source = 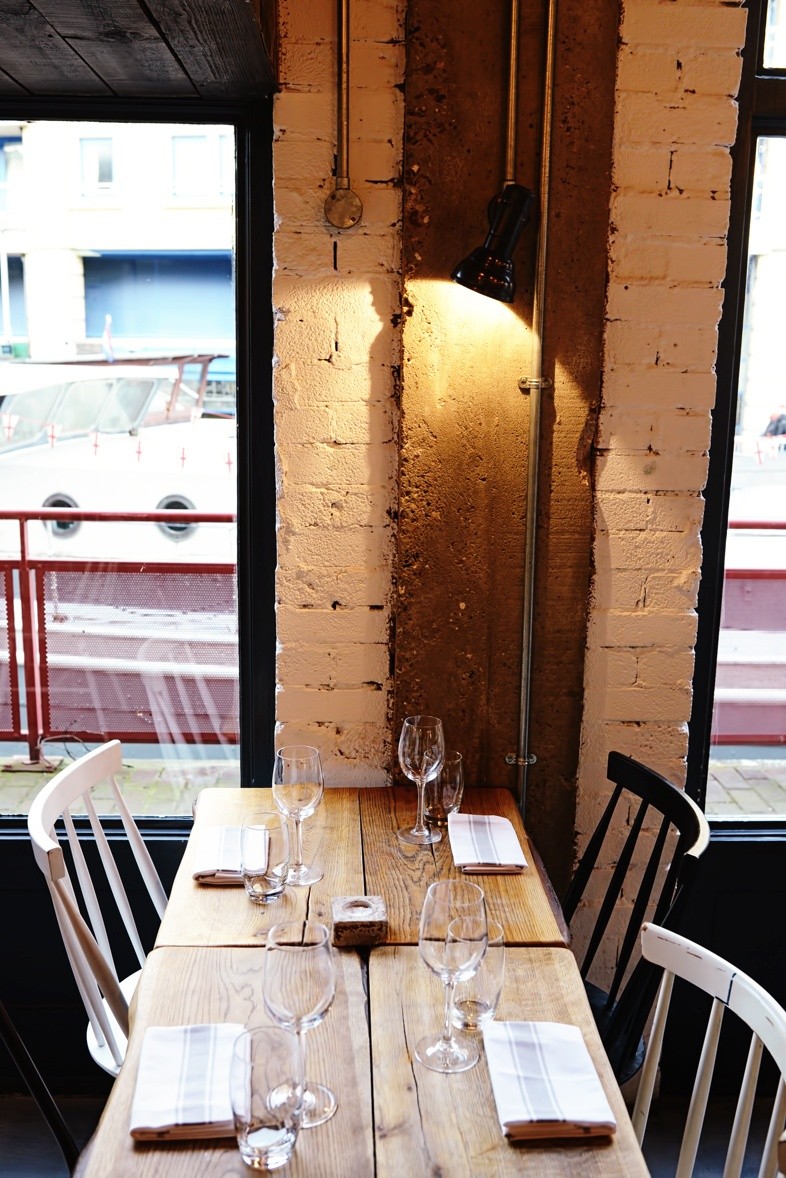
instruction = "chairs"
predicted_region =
[629,921,786,1178]
[562,751,712,1088]
[25,740,169,1081]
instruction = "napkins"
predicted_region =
[478,1020,617,1142]
[193,824,271,885]
[448,812,528,874]
[130,1022,250,1142]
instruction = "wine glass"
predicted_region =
[270,745,326,887]
[411,880,489,1074]
[261,919,339,1129]
[397,715,445,845]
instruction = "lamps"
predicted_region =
[448,184,535,304]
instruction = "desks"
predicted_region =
[69,789,648,1178]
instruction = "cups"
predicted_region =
[229,1026,305,1172]
[444,917,506,1032]
[240,812,290,905]
[419,747,466,827]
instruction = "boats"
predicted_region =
[0,313,786,746]
